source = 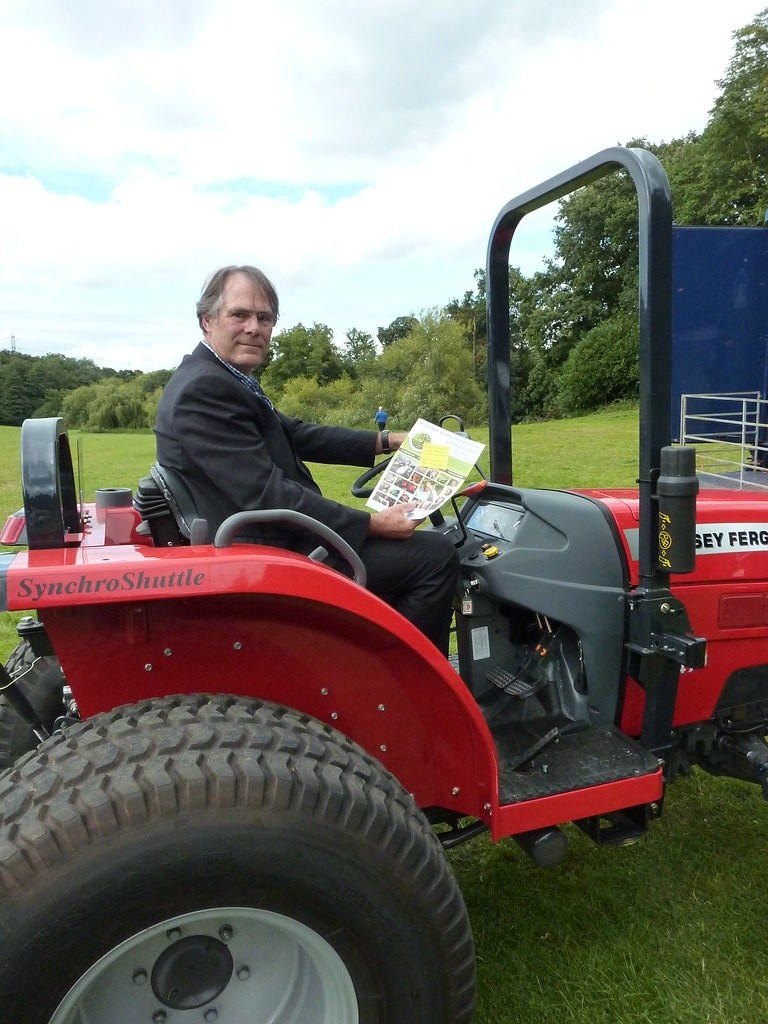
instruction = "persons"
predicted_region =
[152,266,462,664]
[374,405,388,432]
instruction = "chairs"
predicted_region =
[135,458,220,545]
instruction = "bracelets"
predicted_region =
[380,429,391,455]
